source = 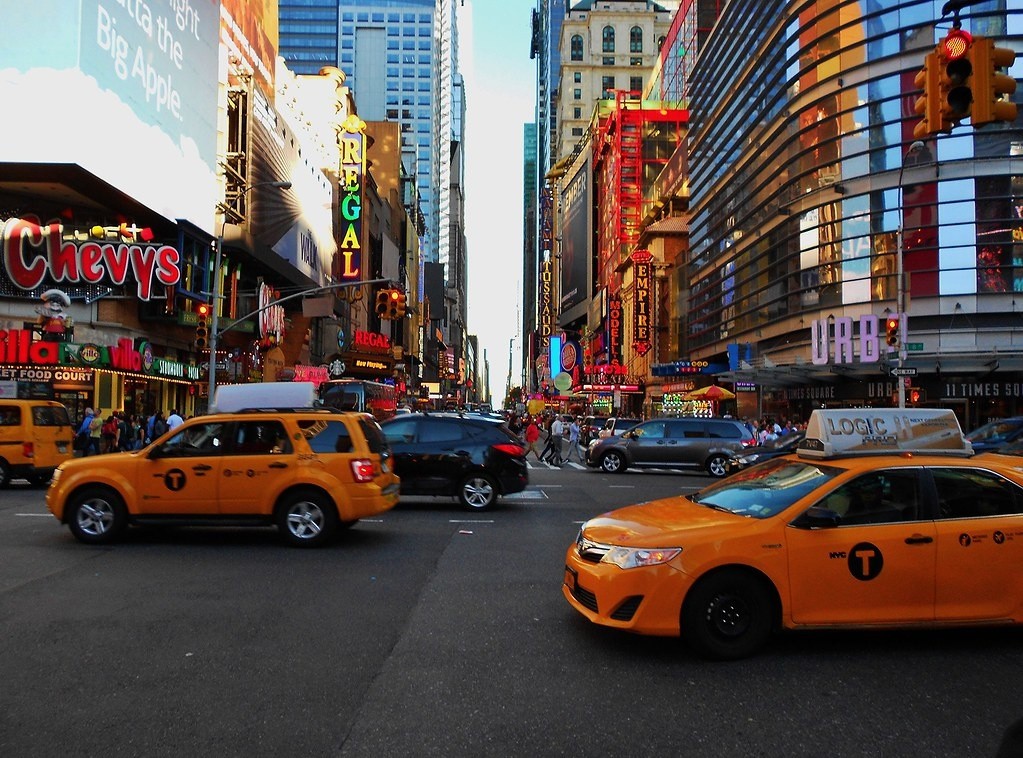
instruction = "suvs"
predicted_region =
[585,417,756,477]
[46,406,400,550]
[380,412,529,511]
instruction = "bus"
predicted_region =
[317,379,397,422]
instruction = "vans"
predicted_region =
[0,398,74,490]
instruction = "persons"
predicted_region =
[967,421,1012,445]
[834,472,906,526]
[70,398,294,459]
[395,397,811,470]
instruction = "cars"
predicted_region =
[724,430,808,476]
[504,409,642,447]
[559,409,1023,659]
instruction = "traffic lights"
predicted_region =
[376,289,408,321]
[913,31,1016,140]
[886,318,899,346]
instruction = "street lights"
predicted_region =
[208,183,292,411]
[898,139,924,410]
